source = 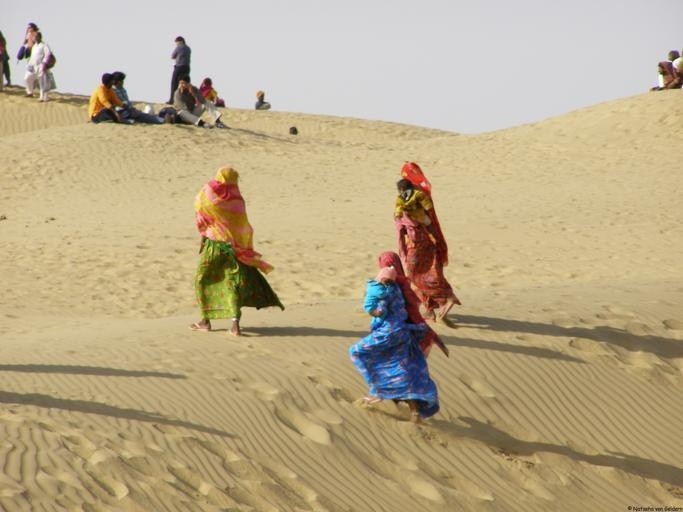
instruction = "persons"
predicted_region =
[189,167,284,335]
[649,50,682,90]
[88,36,231,129]
[255,89,270,110]
[349,161,462,421]
[0,21,55,102]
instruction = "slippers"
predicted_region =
[408,412,422,422]
[420,312,434,319]
[186,323,209,331]
[362,396,381,404]
[225,327,241,335]
[439,297,459,318]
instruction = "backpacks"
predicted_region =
[46,53,55,69]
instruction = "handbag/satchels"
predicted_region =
[37,72,56,93]
